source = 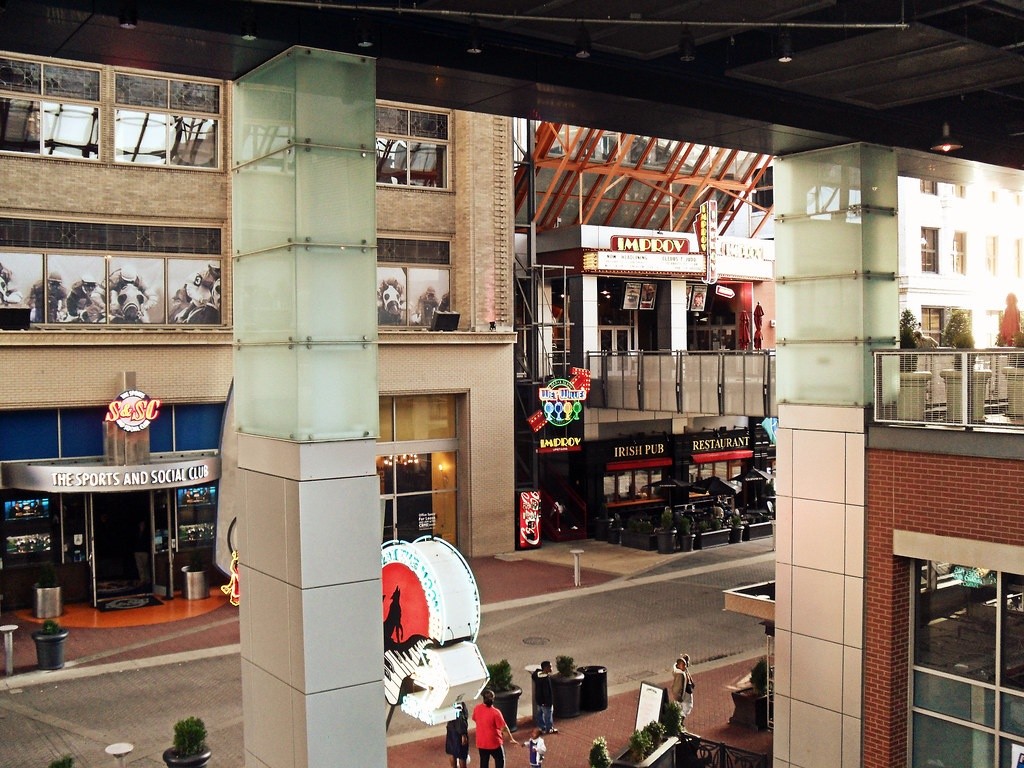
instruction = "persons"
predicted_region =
[915,330,939,349]
[171,260,220,321]
[445,702,470,768]
[0,262,159,323]
[522,727,547,768]
[672,657,693,726]
[684,504,694,515]
[472,689,520,768]
[377,276,450,326]
[532,660,558,733]
[714,502,724,518]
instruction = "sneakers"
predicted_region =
[547,728,558,734]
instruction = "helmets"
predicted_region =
[48,272,61,281]
[120,269,137,282]
[426,287,435,293]
[81,274,98,282]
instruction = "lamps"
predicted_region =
[239,2,258,40]
[726,34,741,69]
[118,0,138,30]
[467,17,482,55]
[676,24,697,64]
[772,26,794,64]
[352,9,376,48]
[573,23,592,60]
[489,320,497,329]
[929,123,966,157]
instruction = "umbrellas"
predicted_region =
[690,475,743,496]
[727,467,776,511]
[753,302,764,348]
[738,306,751,349]
[643,477,706,507]
[1000,292,1021,346]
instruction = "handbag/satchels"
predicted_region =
[686,684,693,694]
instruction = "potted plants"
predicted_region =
[744,514,773,540]
[162,716,212,768]
[592,501,624,544]
[620,519,656,551]
[897,308,932,426]
[480,657,525,733]
[729,514,745,543]
[31,562,63,619]
[695,517,731,550]
[937,307,995,423]
[656,510,678,554]
[181,547,210,600]
[676,516,696,552]
[610,701,702,768]
[551,654,585,719]
[29,617,70,671]
[1002,330,1024,417]
[728,656,775,732]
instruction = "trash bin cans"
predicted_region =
[578,664,607,712]
